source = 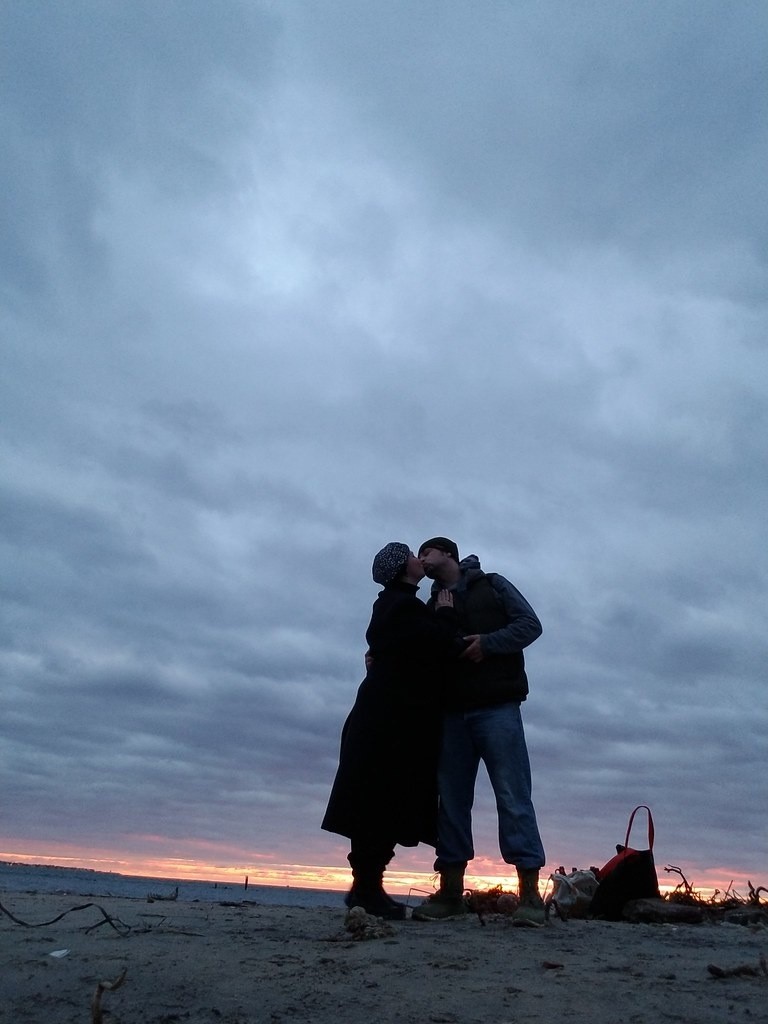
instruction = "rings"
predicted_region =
[445,599,450,601]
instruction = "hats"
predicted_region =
[372,542,410,587]
[418,537,460,563]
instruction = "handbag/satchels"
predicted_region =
[544,870,600,920]
[589,806,660,913]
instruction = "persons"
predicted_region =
[365,538,549,927]
[319,542,456,920]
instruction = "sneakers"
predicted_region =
[413,888,467,922]
[512,896,547,927]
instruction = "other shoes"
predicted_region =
[345,873,406,920]
